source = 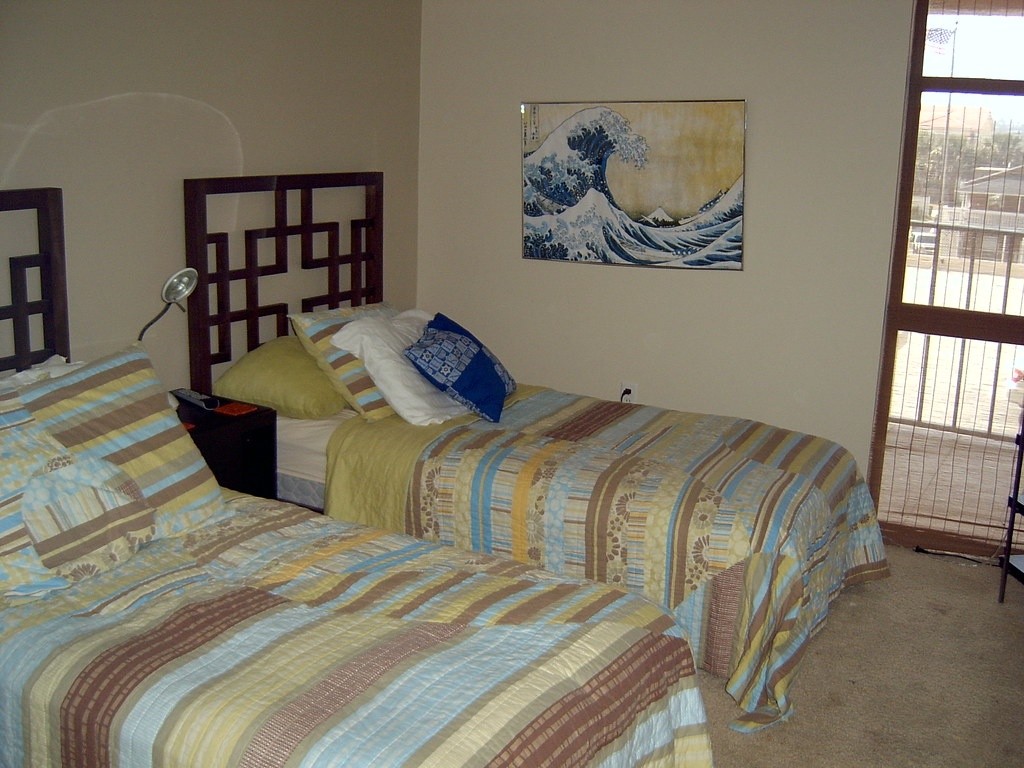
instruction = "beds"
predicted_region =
[184,171,891,734]
[0,187,715,768]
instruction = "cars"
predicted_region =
[910,232,935,255]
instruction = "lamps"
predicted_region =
[137,267,199,342]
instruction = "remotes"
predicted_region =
[173,388,220,410]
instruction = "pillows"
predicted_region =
[402,312,517,423]
[330,308,473,426]
[213,336,349,421]
[284,301,398,423]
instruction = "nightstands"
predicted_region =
[170,387,277,502]
